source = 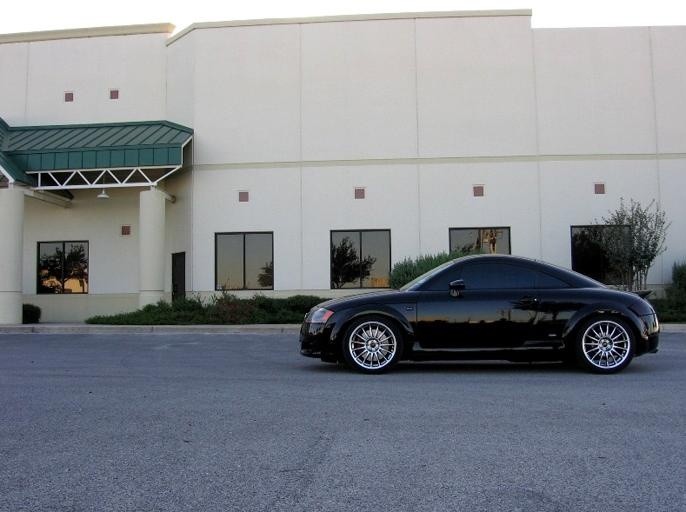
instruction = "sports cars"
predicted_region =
[297,253,663,374]
